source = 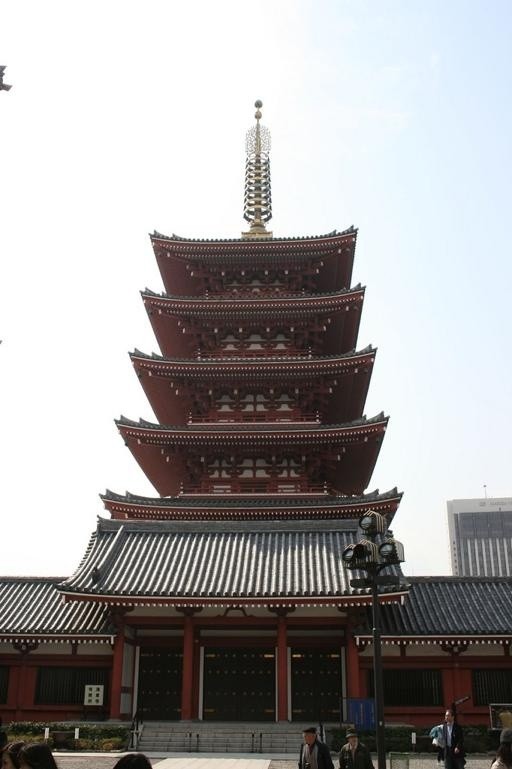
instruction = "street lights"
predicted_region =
[342,510,405,769]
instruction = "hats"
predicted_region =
[302,726,317,735]
[345,727,359,738]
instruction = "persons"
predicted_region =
[435,721,446,767]
[498,710,511,769]
[0,730,59,769]
[491,743,511,769]
[338,728,375,769]
[297,727,334,769]
[441,710,464,769]
[112,753,152,768]
